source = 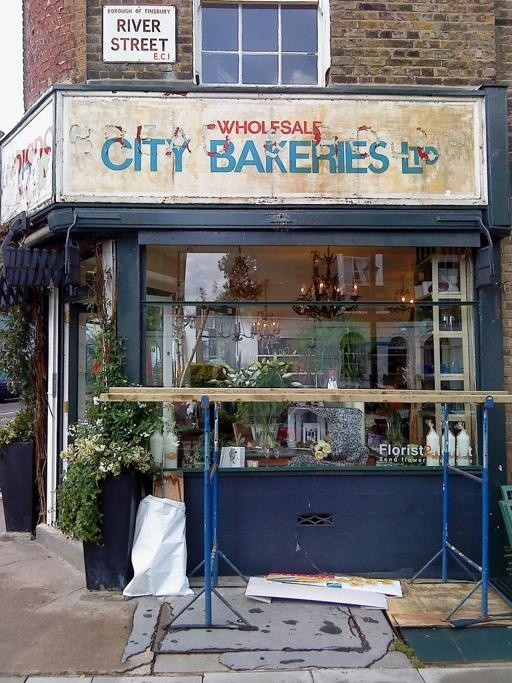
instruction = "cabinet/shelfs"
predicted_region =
[414,252,472,449]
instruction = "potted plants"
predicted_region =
[2,292,36,532]
[303,320,362,388]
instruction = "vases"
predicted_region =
[385,414,404,443]
[81,461,143,592]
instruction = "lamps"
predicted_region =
[218,245,362,343]
[385,272,416,314]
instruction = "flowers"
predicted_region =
[37,293,167,549]
[206,348,302,447]
[371,402,405,444]
